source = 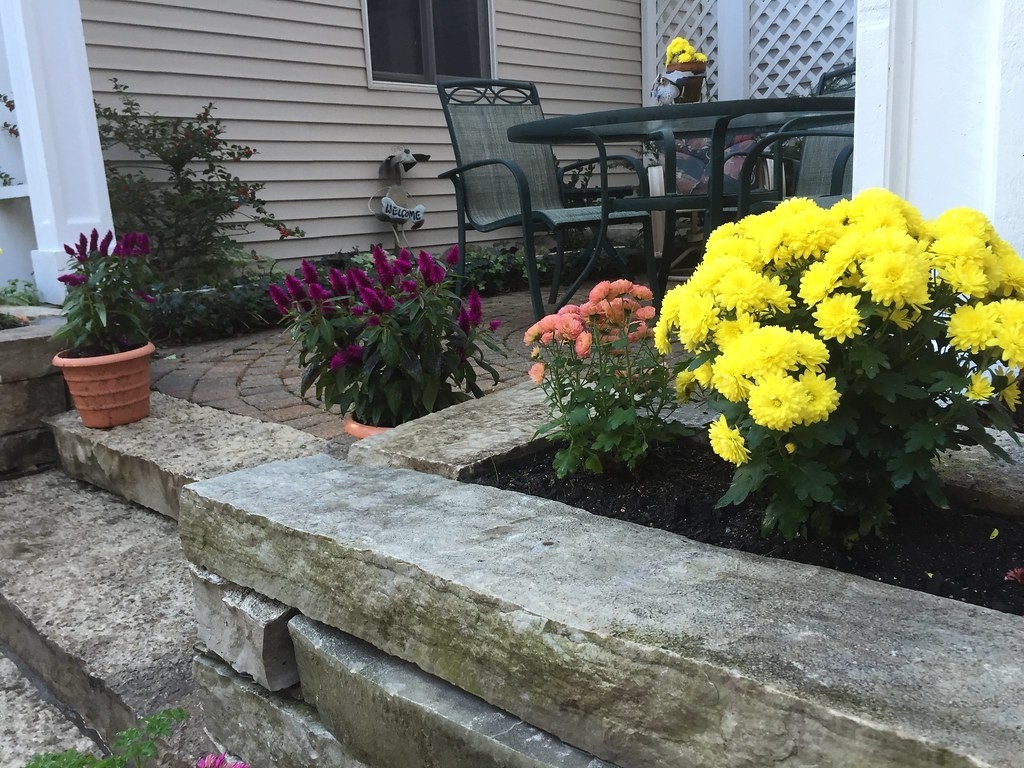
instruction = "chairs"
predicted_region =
[436,77,659,329]
[701,62,856,254]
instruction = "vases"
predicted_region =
[344,392,473,440]
[650,62,706,104]
[52,341,156,428]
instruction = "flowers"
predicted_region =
[267,241,512,430]
[48,228,159,356]
[665,37,707,66]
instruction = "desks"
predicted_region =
[507,97,853,328]
